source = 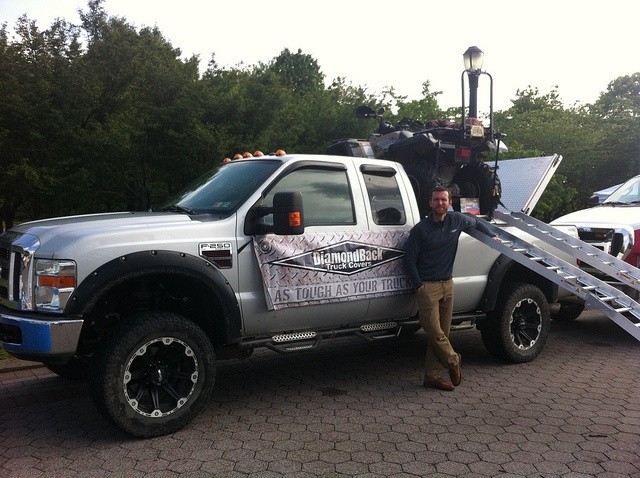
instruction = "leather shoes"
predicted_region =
[450,353,461,385]
[424,378,455,391]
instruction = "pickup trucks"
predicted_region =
[552,175,639,324]
[0,150,586,438]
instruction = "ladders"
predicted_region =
[464,213,640,344]
[493,210,639,292]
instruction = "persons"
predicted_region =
[402,185,501,393]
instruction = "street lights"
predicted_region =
[462,46,494,142]
